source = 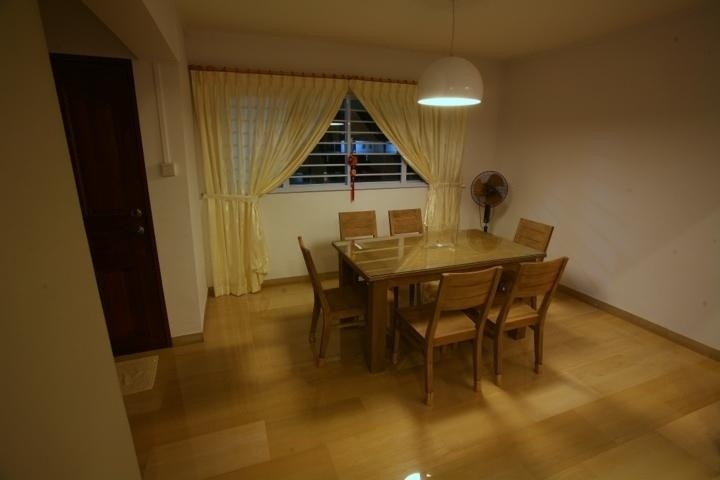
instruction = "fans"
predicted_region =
[470,170,509,231]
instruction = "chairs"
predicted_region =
[389,265,502,407]
[499,218,555,313]
[338,208,443,307]
[297,236,400,365]
[447,256,569,386]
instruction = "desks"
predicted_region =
[332,228,547,375]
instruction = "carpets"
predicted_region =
[116,353,159,396]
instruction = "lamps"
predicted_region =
[415,1,485,109]
